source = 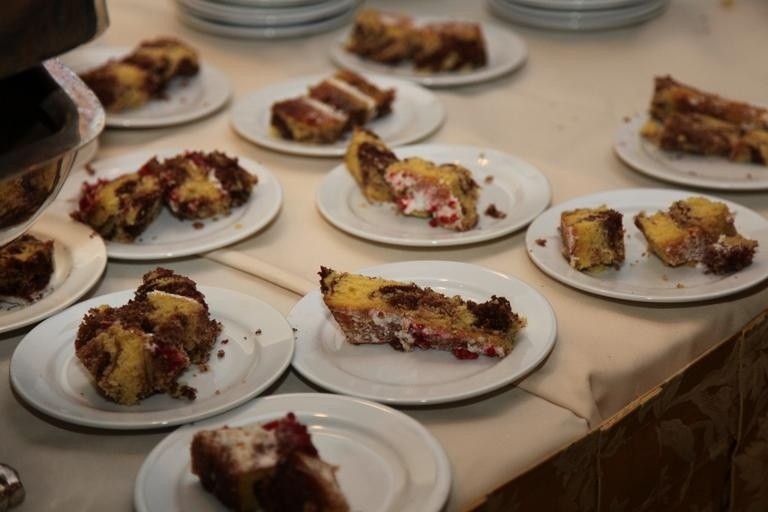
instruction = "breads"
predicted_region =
[0,234,54,301]
[344,122,505,233]
[344,10,488,73]
[68,147,257,243]
[271,65,396,146]
[189,412,349,510]
[639,72,764,178]
[78,34,199,114]
[316,265,527,362]
[560,204,625,272]
[633,196,759,276]
[73,266,228,405]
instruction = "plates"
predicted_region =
[330,18,532,91]
[230,72,447,158]
[287,259,559,407]
[175,2,358,41]
[0,211,107,335]
[54,45,231,132]
[8,285,298,430]
[525,188,768,305]
[491,1,666,34]
[616,101,766,191]
[59,148,285,260]
[314,145,552,251]
[133,389,451,509]
